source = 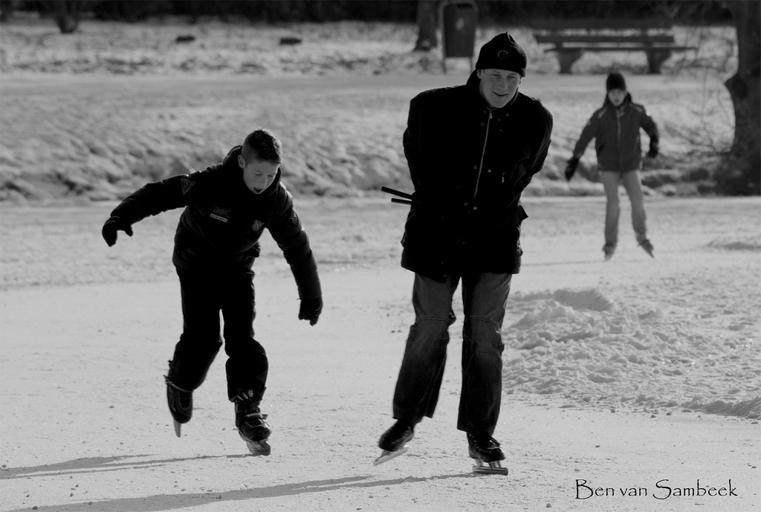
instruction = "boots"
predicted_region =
[602,243,614,253]
[641,240,652,251]
[235,403,271,439]
[379,423,414,451]
[469,435,503,461]
[167,382,192,422]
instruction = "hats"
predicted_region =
[607,73,625,90]
[478,33,526,77]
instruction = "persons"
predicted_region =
[379,33,552,460]
[564,72,659,253]
[102,129,322,438]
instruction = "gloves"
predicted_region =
[647,149,657,158]
[299,297,321,325]
[565,160,575,180]
[102,216,132,246]
